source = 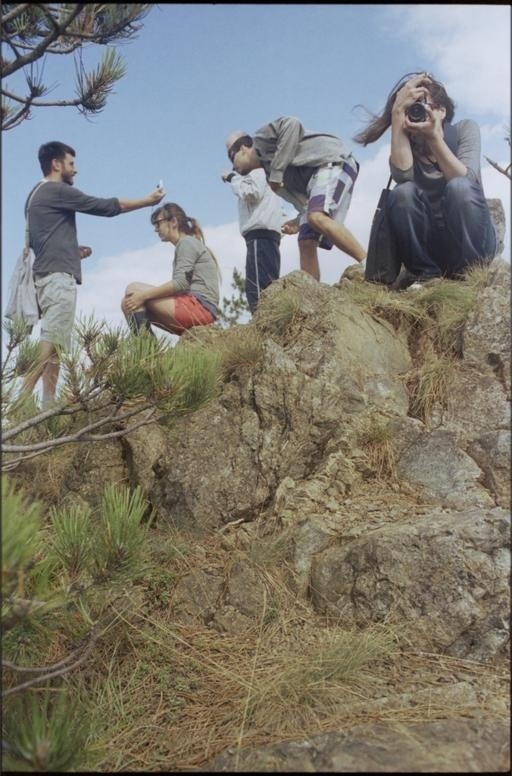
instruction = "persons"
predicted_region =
[8,139,167,420]
[225,115,368,284]
[220,168,286,316]
[352,71,496,293]
[121,201,220,355]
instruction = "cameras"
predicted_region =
[407,95,434,122]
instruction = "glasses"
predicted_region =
[152,216,171,228]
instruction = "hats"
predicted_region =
[225,130,247,151]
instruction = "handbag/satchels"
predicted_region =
[364,189,401,284]
[4,248,39,326]
